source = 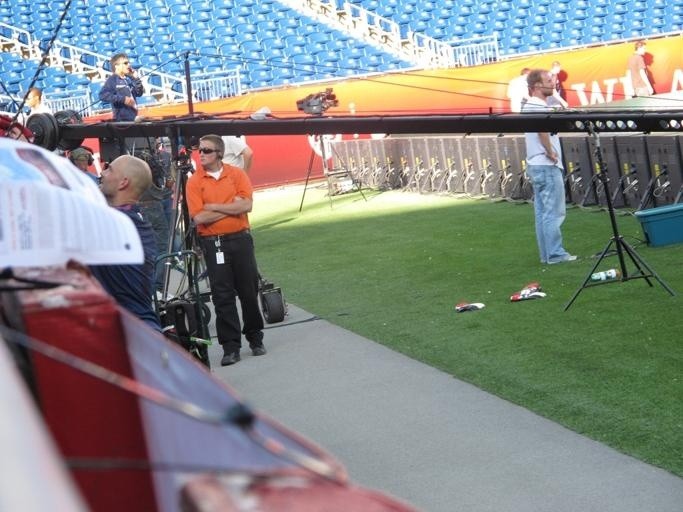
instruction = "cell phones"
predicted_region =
[129,68,132,73]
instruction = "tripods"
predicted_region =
[300,135,366,210]
[564,124,676,312]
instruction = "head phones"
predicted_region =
[69,146,95,164]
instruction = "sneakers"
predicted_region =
[540,255,578,265]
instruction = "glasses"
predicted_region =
[199,147,220,154]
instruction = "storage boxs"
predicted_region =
[634,203,683,247]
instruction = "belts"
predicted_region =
[203,230,249,241]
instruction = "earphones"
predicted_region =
[218,156,222,160]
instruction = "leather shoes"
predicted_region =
[222,351,240,366]
[252,338,266,355]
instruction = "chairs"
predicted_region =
[2,1,420,100]
[1,49,161,113]
[321,0,683,66]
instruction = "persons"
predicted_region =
[98,52,143,119]
[627,41,654,98]
[518,67,577,266]
[7,88,176,334]
[506,68,531,114]
[183,133,266,366]
[217,133,253,173]
[543,60,571,109]
